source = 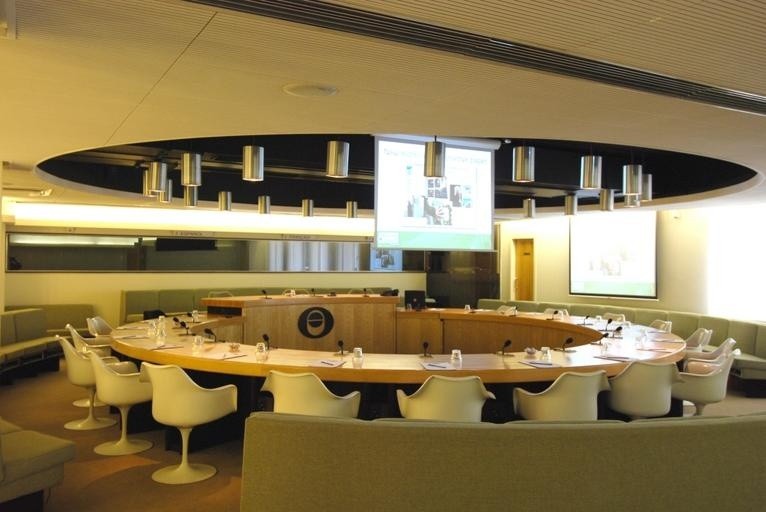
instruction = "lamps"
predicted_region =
[142,135,270,214]
[346,201,357,219]
[423,134,446,178]
[511,139,652,218]
[302,199,313,217]
[324,134,350,179]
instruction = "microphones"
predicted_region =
[336,339,347,356]
[612,326,623,337]
[262,289,269,297]
[311,286,315,295]
[562,336,575,351]
[174,316,181,325]
[605,318,613,330]
[204,327,217,342]
[180,320,188,332]
[598,333,609,341]
[421,341,430,357]
[262,332,271,350]
[362,287,368,295]
[501,339,513,355]
[583,315,589,324]
[550,311,559,319]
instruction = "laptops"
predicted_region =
[405,288,428,308]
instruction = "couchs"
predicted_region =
[477,298,766,384]
[0,304,96,512]
[237,409,765,510]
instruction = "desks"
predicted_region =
[110,292,689,450]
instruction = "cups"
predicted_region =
[539,347,550,363]
[406,304,411,311]
[600,346,608,359]
[557,311,563,320]
[451,349,462,363]
[148,310,267,360]
[351,348,364,368]
[595,316,601,325]
[464,304,470,313]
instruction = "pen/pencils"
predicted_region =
[530,362,552,365]
[608,356,629,359]
[321,361,333,365]
[428,363,446,368]
